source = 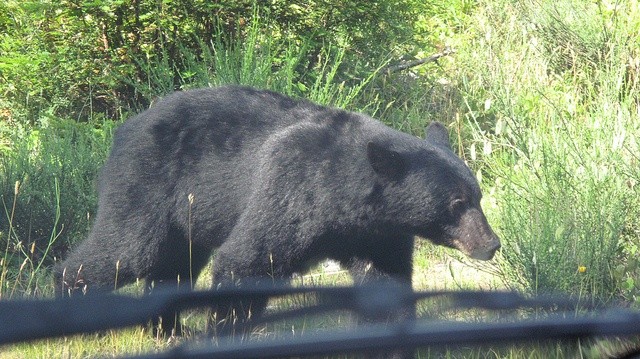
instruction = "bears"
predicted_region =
[48,84,502,358]
